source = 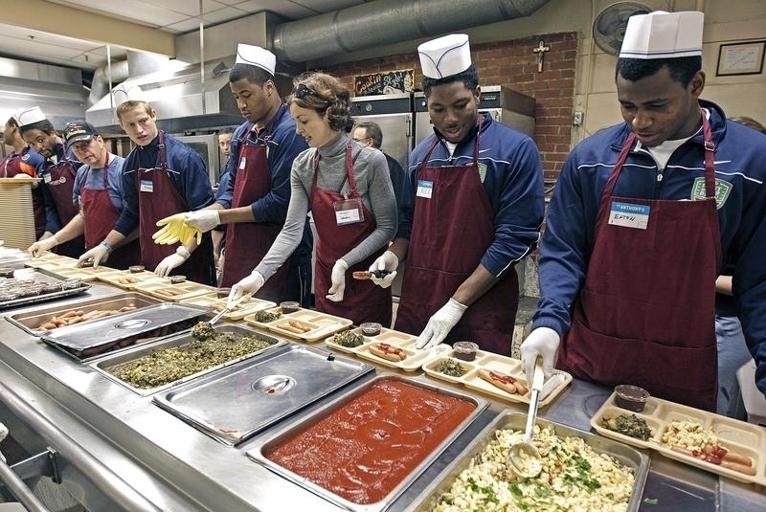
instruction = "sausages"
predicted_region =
[210,302,239,314]
[35,301,189,359]
[476,369,528,395]
[118,276,135,284]
[370,341,409,362]
[678,446,757,475]
[280,321,310,335]
[156,288,180,299]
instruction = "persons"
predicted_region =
[15,106,86,260]
[152,43,312,306]
[227,71,398,330]
[368,32,546,357]
[0,109,46,241]
[714,116,766,419]
[27,121,141,271]
[519,11,766,415]
[211,126,236,288]
[73,85,217,288]
[351,120,406,210]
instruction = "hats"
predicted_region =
[114,82,146,107]
[618,9,707,60]
[235,43,277,77]
[14,105,47,127]
[62,120,99,152]
[417,33,472,80]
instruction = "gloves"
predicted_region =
[152,210,220,246]
[325,260,346,302]
[518,327,560,389]
[227,271,264,310]
[29,236,58,258]
[414,298,468,350]
[368,250,399,289]
[154,245,192,278]
[76,241,112,268]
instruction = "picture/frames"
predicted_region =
[716,40,766,76]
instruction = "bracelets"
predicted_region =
[101,241,113,252]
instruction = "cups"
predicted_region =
[452,339,480,361]
[78,259,95,268]
[360,321,382,337]
[216,287,235,299]
[169,274,185,284]
[280,300,300,314]
[616,382,651,412]
[129,265,145,274]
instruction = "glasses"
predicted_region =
[291,83,327,101]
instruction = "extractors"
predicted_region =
[83,12,307,140]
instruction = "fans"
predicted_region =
[592,0,653,56]
